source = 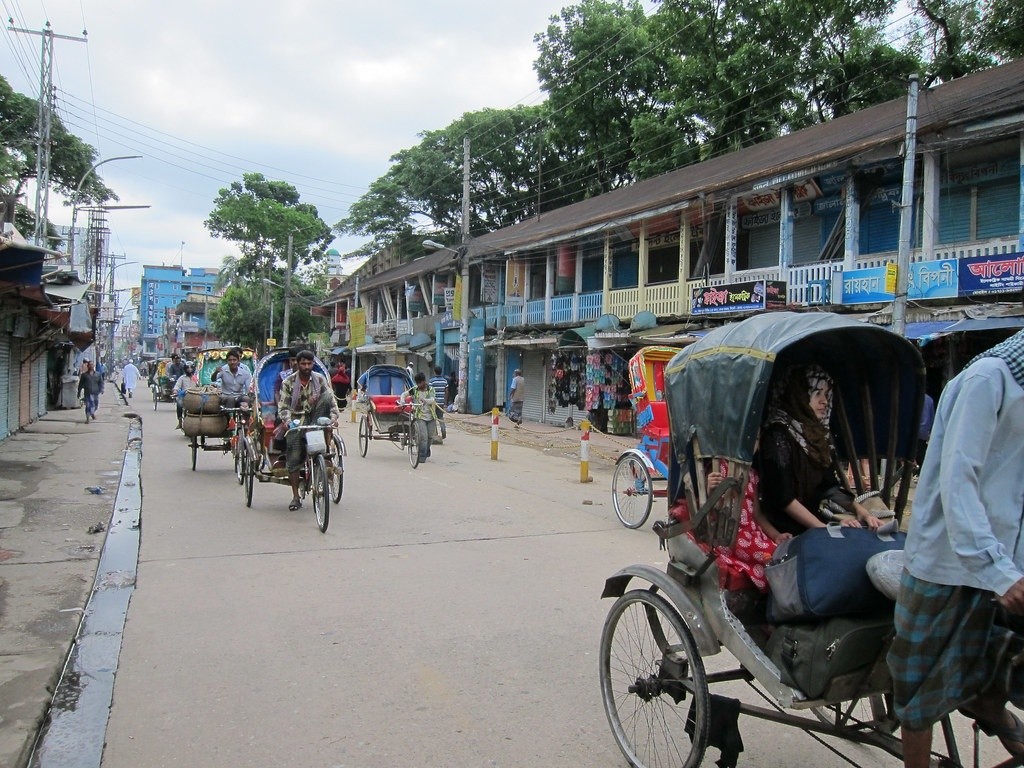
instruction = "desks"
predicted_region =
[644,401,670,438]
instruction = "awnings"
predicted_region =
[886,314,1024,344]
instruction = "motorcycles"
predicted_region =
[170,390,193,437]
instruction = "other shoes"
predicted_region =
[289,500,301,508]
[419,456,426,463]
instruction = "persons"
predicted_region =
[428,366,449,439]
[399,372,436,464]
[507,369,525,428]
[99,347,350,512]
[668,425,794,634]
[406,362,415,381]
[752,361,884,537]
[887,323,1024,768]
[77,361,104,424]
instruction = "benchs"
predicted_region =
[368,395,412,413]
[259,402,275,447]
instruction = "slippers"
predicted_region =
[958,704,1024,766]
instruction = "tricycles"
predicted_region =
[357,364,425,469]
[597,307,1024,768]
[610,345,671,530]
[150,356,180,410]
[137,360,148,378]
[231,347,347,533]
[187,340,255,485]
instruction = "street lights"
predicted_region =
[421,240,469,413]
[262,278,289,347]
